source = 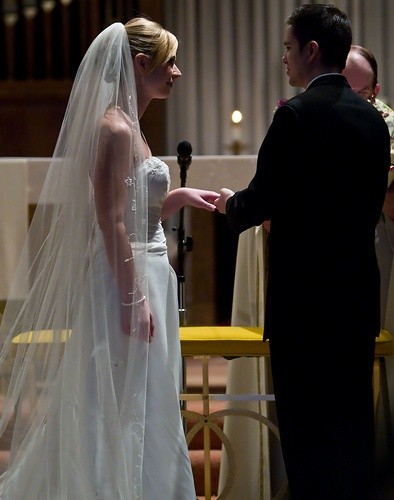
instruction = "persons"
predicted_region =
[213,3,394,500]
[0,16,220,500]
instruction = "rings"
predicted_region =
[132,328,137,333]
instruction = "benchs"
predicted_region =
[9,326,394,500]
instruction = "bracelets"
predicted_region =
[121,296,145,308]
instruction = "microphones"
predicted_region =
[176,141,193,179]
